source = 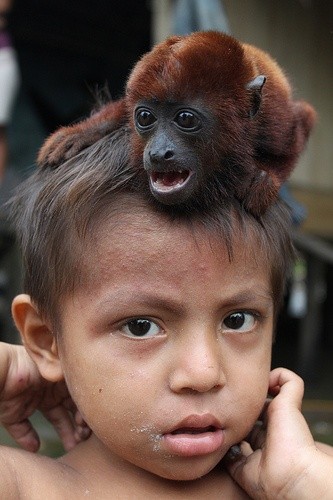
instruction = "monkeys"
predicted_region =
[38,30,316,216]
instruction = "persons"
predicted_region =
[0,122,333,500]
[0,1,20,188]
